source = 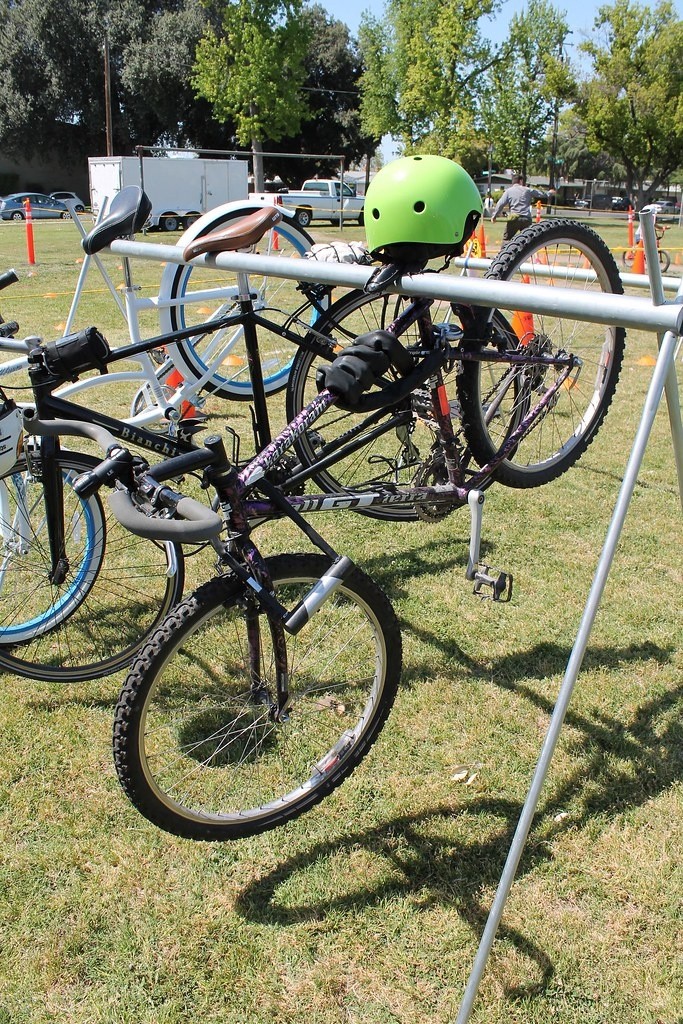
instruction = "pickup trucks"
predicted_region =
[248,179,366,226]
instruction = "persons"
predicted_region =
[491,174,556,257]
[485,196,494,207]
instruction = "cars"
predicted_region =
[47,192,85,212]
[1,192,72,221]
[651,201,680,214]
[612,197,631,211]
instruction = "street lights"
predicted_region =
[546,29,573,214]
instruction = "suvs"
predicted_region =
[575,193,613,211]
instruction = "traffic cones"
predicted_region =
[541,254,547,264]
[504,273,538,342]
[630,241,645,277]
[463,223,487,260]
[673,253,682,265]
[583,258,592,270]
[160,342,207,423]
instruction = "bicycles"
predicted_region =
[623,224,671,272]
[0,185,628,841]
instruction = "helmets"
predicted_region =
[0,399,23,475]
[363,154,482,259]
[643,205,657,216]
